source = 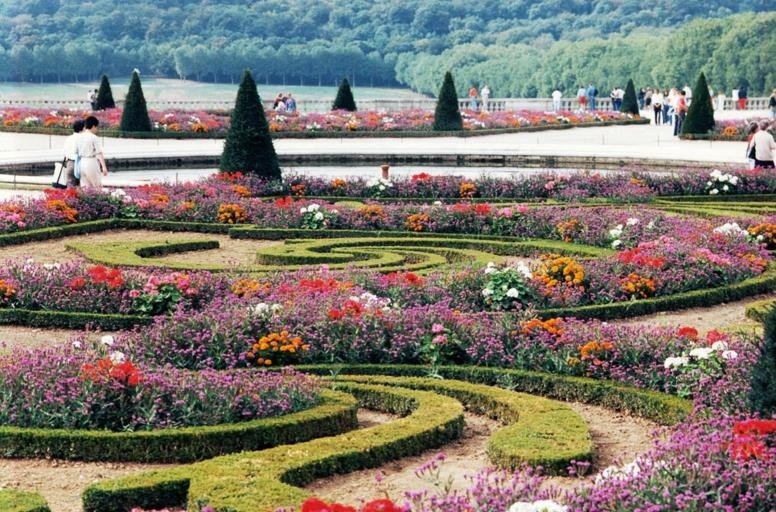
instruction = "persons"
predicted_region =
[768,88,776,118]
[746,120,776,169]
[470,84,490,112]
[273,92,296,112]
[87,89,98,110]
[732,86,747,110]
[552,83,714,136]
[65,116,108,190]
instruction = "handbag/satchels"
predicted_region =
[51,157,67,189]
[748,135,756,160]
